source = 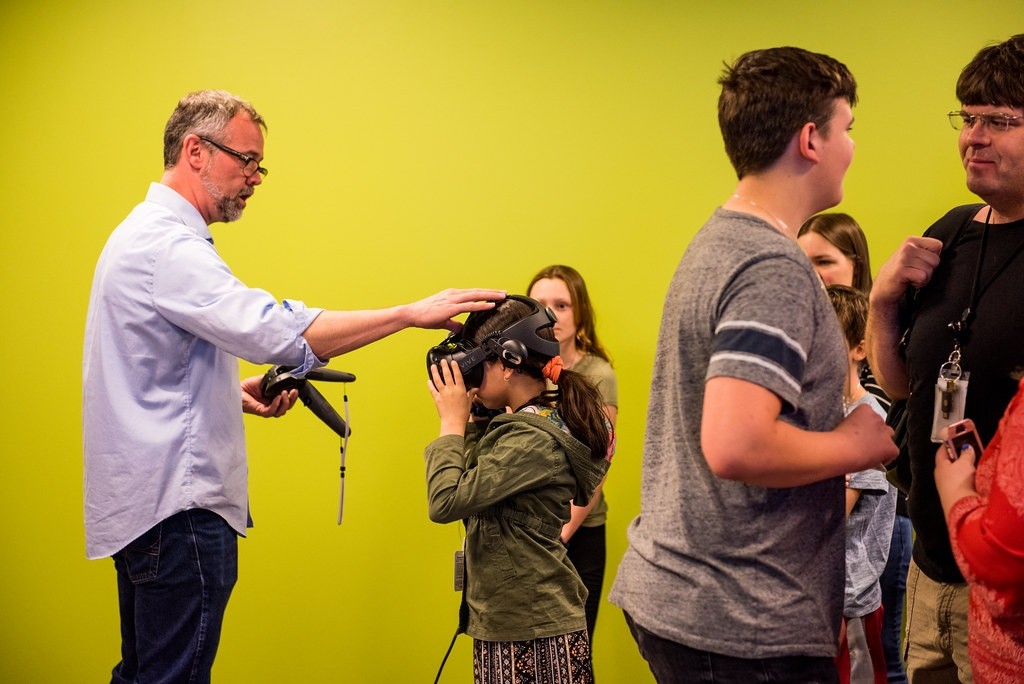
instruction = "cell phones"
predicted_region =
[939,418,985,468]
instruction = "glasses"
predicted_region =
[181,136,268,182]
[947,110,1024,135]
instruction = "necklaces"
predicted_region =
[845,381,860,408]
[732,193,852,398]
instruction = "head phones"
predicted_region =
[458,294,560,372]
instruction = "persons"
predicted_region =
[862,32,1024,684]
[85,89,509,684]
[797,213,914,684]
[607,47,901,684]
[424,294,614,684]
[935,376,1024,684]
[525,265,619,641]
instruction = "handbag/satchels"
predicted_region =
[880,202,986,500]
